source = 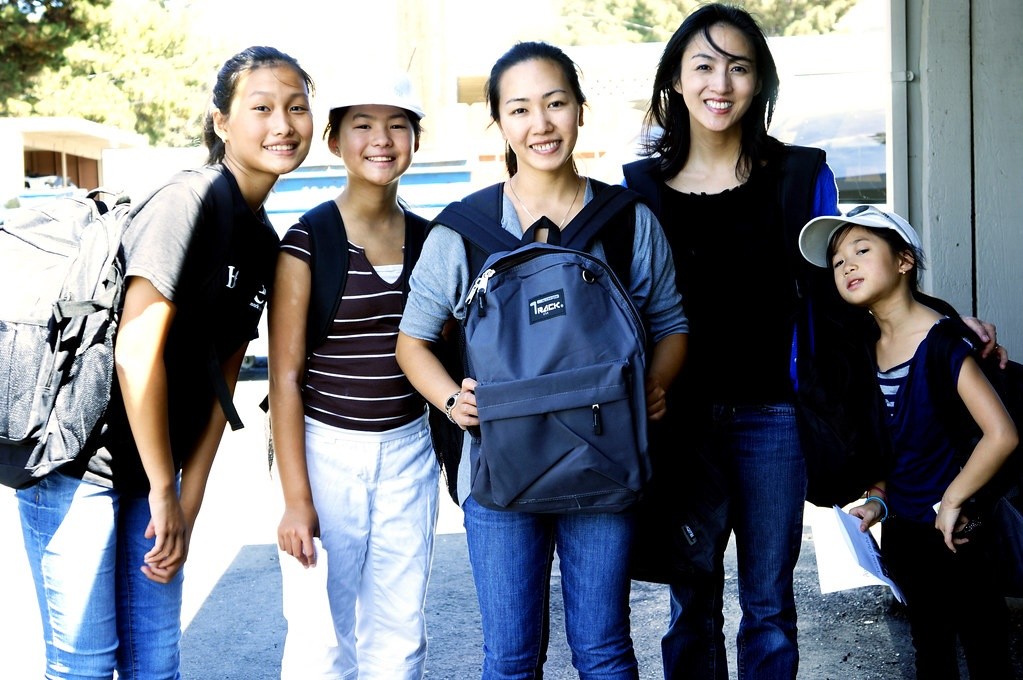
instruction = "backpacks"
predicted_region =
[0,164,220,490]
[425,184,653,514]
[926,319,1023,505]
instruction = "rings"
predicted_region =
[994,344,1000,352]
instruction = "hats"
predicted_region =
[799,204,924,282]
[324,61,425,122]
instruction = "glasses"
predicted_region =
[843,205,912,244]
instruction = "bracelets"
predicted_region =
[872,485,887,498]
[865,496,888,522]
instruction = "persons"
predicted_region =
[394,39,687,680]
[267,78,459,680]
[793,204,1023,680]
[613,5,1007,680]
[11,48,318,680]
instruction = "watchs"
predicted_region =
[445,392,461,425]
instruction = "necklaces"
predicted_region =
[509,177,581,230]
